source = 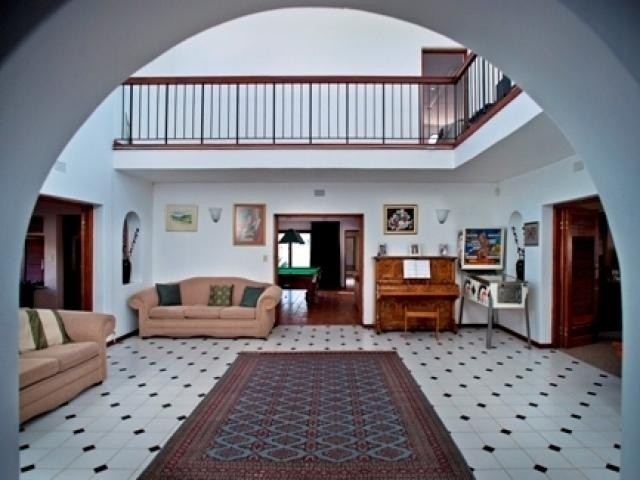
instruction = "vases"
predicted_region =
[123,259,130,283]
[516,260,524,281]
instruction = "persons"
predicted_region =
[463,279,472,297]
[477,286,488,304]
[476,229,496,265]
[240,213,262,241]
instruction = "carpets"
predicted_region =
[136,351,475,480]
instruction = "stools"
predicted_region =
[404,306,440,340]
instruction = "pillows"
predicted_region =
[208,283,234,306]
[19,307,72,354]
[156,283,182,306]
[240,287,264,307]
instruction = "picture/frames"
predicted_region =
[524,221,538,246]
[166,204,197,231]
[384,204,418,234]
[232,203,266,246]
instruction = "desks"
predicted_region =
[458,273,531,350]
[278,267,322,308]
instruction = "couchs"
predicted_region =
[128,277,283,341]
[19,309,116,425]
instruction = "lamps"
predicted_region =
[278,228,305,266]
[436,209,450,224]
[209,208,222,223]
